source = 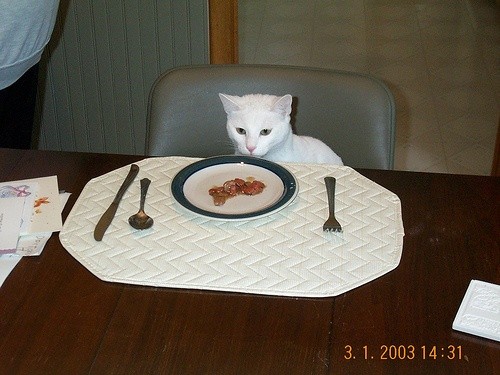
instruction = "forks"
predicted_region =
[323,177,342,233]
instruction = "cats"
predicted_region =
[218,92,343,164]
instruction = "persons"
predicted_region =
[0,0,59,149]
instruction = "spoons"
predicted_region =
[128,178,153,230]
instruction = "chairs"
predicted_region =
[143,63,394,170]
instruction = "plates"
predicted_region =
[170,154,299,222]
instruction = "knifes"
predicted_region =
[94,164,139,241]
